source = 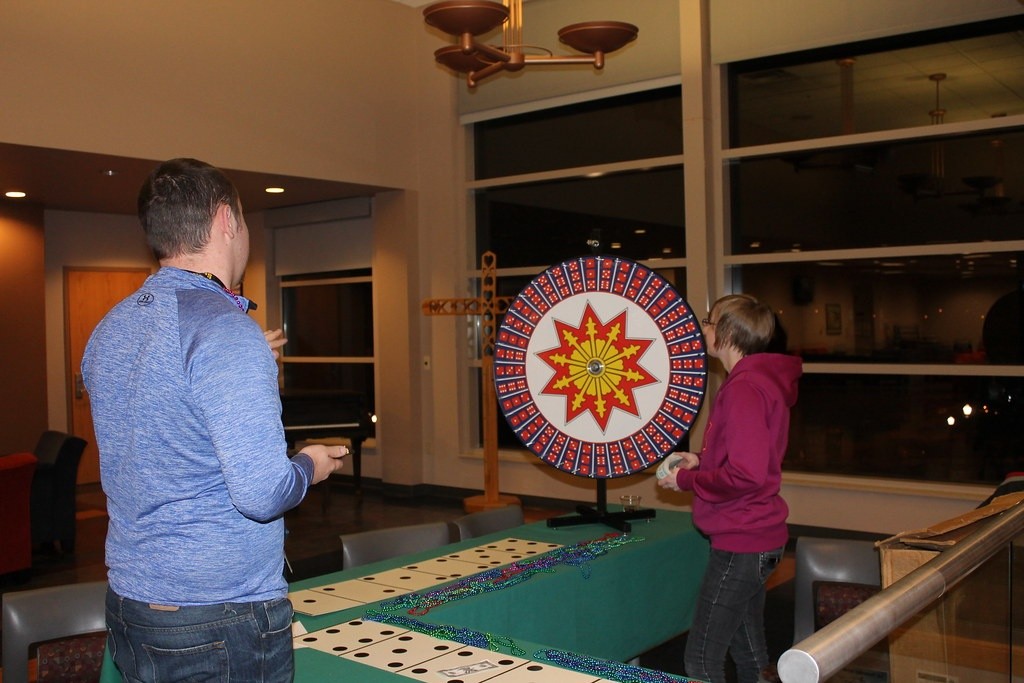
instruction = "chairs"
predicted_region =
[340,506,525,570]
[2,579,109,683]
[794,537,890,683]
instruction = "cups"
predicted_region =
[620,495,642,513]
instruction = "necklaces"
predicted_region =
[224,288,245,312]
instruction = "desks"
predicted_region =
[100,502,714,682]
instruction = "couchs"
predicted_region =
[0,431,87,586]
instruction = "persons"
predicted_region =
[81,158,346,683]
[658,294,802,683]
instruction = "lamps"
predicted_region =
[423,0,639,88]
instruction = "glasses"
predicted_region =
[702,319,716,327]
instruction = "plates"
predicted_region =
[287,446,354,459]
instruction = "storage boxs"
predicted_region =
[875,490,1024,683]
